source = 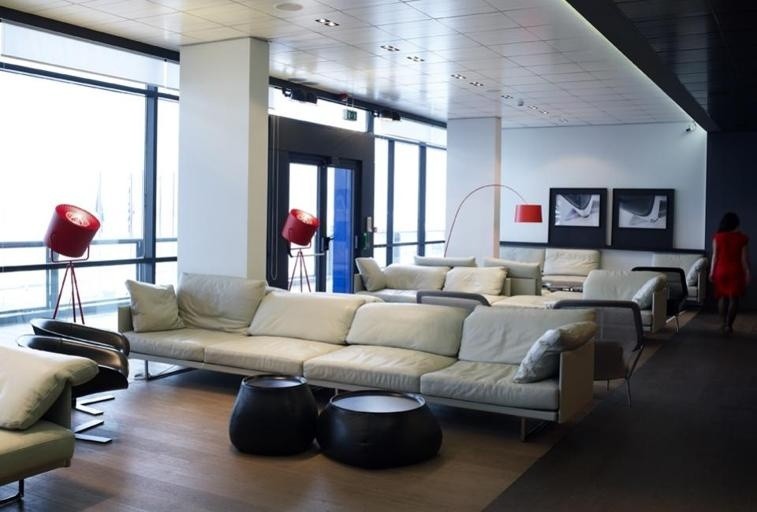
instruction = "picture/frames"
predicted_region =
[611,188,677,250]
[548,187,608,249]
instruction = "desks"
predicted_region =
[480,314,757,512]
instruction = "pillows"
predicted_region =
[0,347,99,431]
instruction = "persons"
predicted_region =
[709,212,750,337]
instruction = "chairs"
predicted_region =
[17,318,131,446]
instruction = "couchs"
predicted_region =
[0,347,74,507]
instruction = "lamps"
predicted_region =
[444,184,542,258]
[282,209,320,292]
[43,204,101,324]
